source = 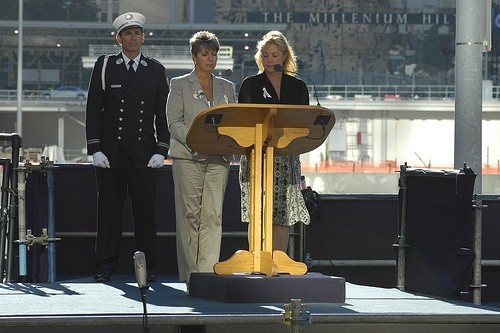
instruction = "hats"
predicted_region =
[113,12,146,35]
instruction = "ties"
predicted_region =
[128,60,135,80]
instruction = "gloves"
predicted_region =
[92,150,110,168]
[147,153,165,168]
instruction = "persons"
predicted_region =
[86,12,171,283]
[238,30,311,253]
[165,31,237,284]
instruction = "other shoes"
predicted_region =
[147,271,155,281]
[94,263,113,281]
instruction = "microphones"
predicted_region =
[133,251,147,291]
[274,64,320,105]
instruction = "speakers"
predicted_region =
[396,163,476,302]
